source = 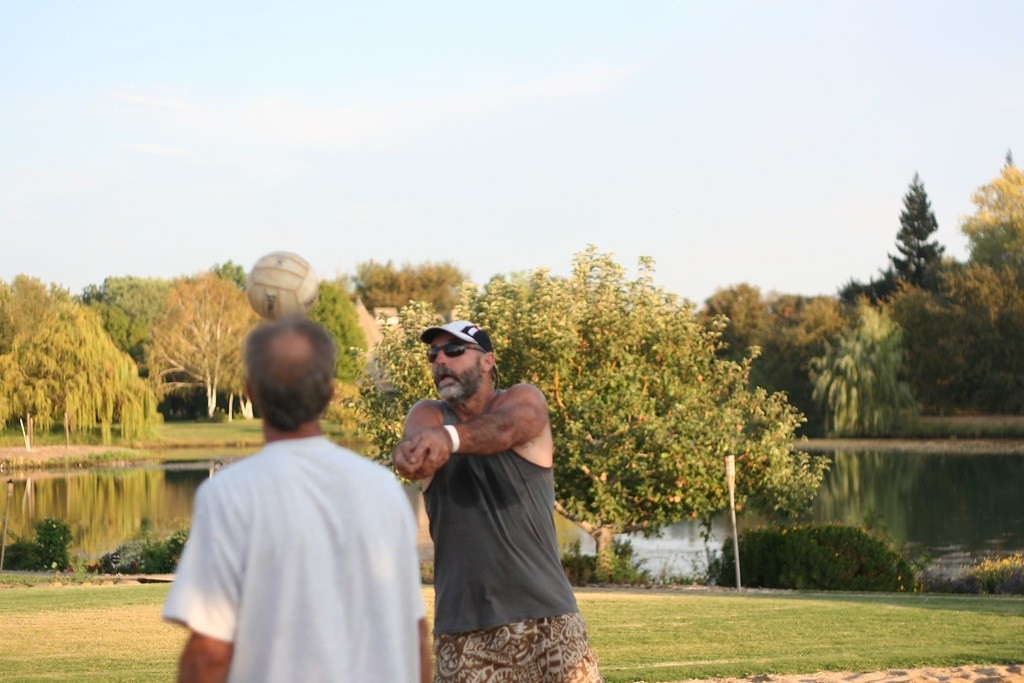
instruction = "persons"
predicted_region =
[391,318,603,683]
[164,314,432,683]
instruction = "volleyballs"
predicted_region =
[245,250,322,322]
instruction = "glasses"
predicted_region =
[426,342,487,363]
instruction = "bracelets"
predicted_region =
[443,425,461,454]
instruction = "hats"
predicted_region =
[419,320,493,354]
